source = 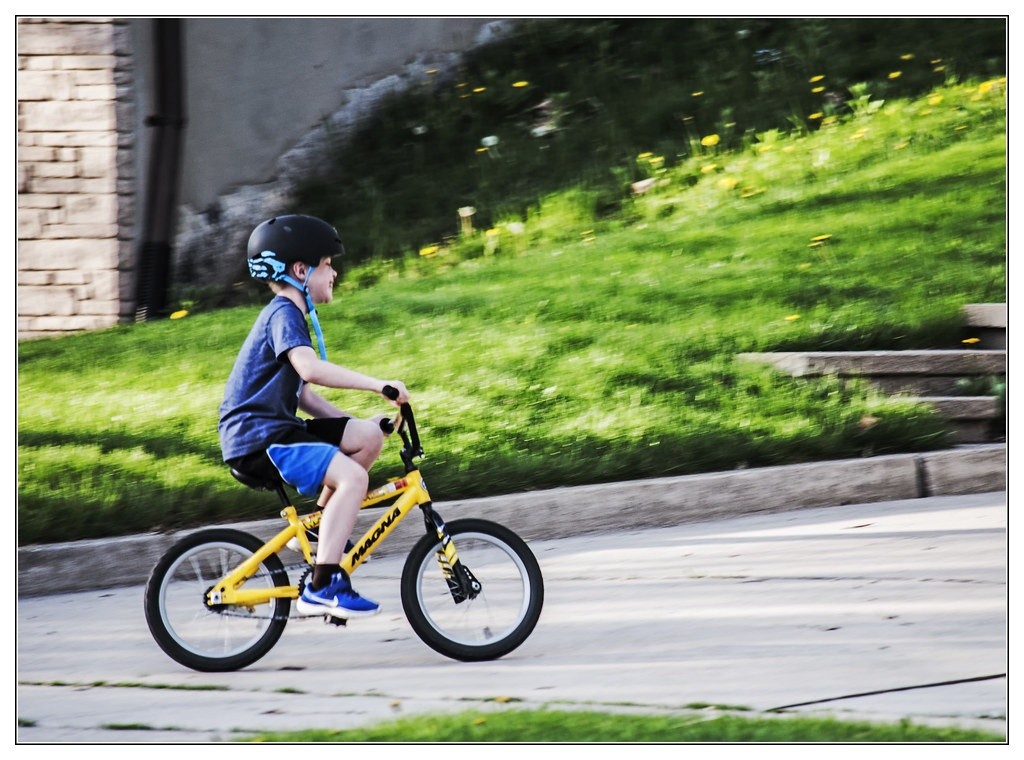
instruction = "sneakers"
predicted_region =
[286,529,373,567]
[295,573,380,620]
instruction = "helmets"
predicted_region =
[244,213,346,279]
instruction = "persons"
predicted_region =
[217,214,411,618]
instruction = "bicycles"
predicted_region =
[142,384,544,675]
